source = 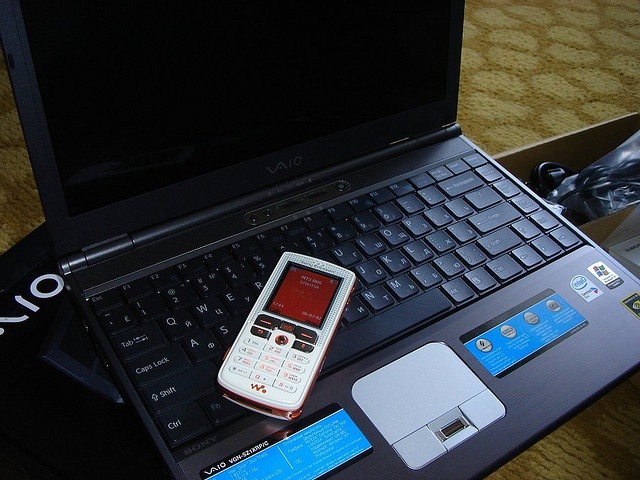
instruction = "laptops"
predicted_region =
[0,0,640,480]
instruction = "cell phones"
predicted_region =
[214,251,357,422]
